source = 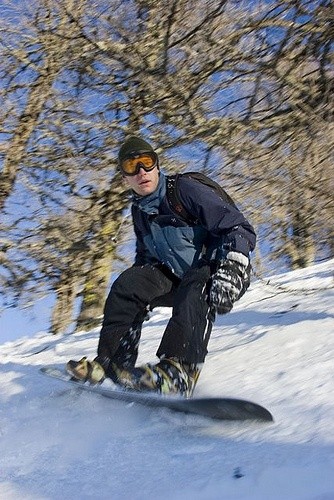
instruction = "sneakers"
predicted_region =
[66,357,132,391]
[118,357,201,399]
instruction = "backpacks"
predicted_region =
[164,172,253,317]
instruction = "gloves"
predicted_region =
[199,250,251,315]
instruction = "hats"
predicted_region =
[118,137,160,178]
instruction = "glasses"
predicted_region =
[119,150,158,176]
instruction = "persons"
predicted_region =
[67,137,256,398]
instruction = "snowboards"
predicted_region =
[38,368,272,422]
